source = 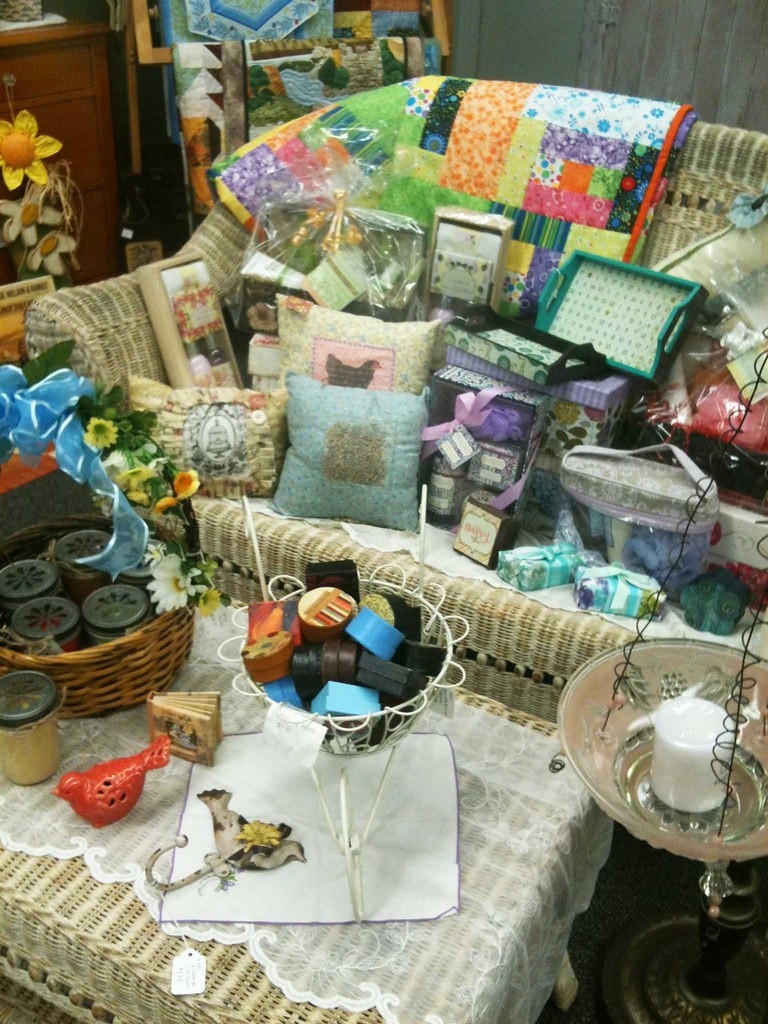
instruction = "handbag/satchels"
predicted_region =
[558,442,720,602]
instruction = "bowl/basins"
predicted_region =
[557,638,768,863]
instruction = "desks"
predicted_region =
[1,0,122,292]
[0,556,611,1024]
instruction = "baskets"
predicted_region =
[0,512,196,718]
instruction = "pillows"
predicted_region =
[128,375,286,499]
[268,366,429,536]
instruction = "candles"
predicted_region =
[649,698,737,816]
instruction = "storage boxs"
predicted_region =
[441,324,669,625]
[704,487,768,613]
[136,252,245,394]
[419,205,514,318]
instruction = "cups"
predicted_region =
[0,559,62,623]
[82,584,150,645]
[12,597,80,650]
[112,539,167,595]
[54,530,111,605]
[0,672,66,785]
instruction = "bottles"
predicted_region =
[116,175,170,274]
[426,410,469,526]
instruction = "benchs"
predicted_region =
[30,75,768,723]
